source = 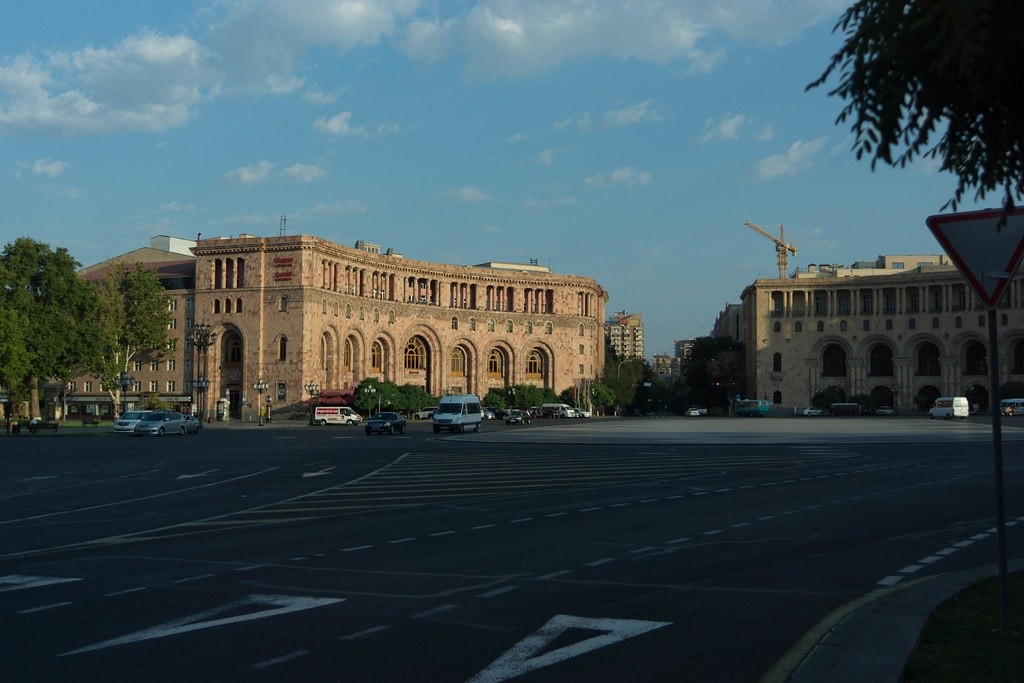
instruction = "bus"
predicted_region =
[1000,398,1024,416]
[734,400,770,418]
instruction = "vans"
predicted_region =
[531,402,590,419]
[428,394,482,434]
[314,406,363,426]
[928,396,969,419]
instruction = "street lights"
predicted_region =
[305,380,320,426]
[192,374,211,421]
[115,371,136,414]
[252,377,269,426]
[618,359,632,379]
[365,384,376,420]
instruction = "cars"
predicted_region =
[111,410,154,434]
[798,407,806,416]
[134,411,186,437]
[181,413,200,434]
[414,406,436,420]
[481,406,519,420]
[803,407,824,417]
[364,412,407,435]
[505,410,531,426]
[684,407,700,417]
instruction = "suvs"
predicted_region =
[876,406,894,416]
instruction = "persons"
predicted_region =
[29,417,37,432]
[182,407,199,420]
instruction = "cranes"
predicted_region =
[740,218,797,278]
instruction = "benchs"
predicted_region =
[28,422,59,434]
[83,420,99,428]
